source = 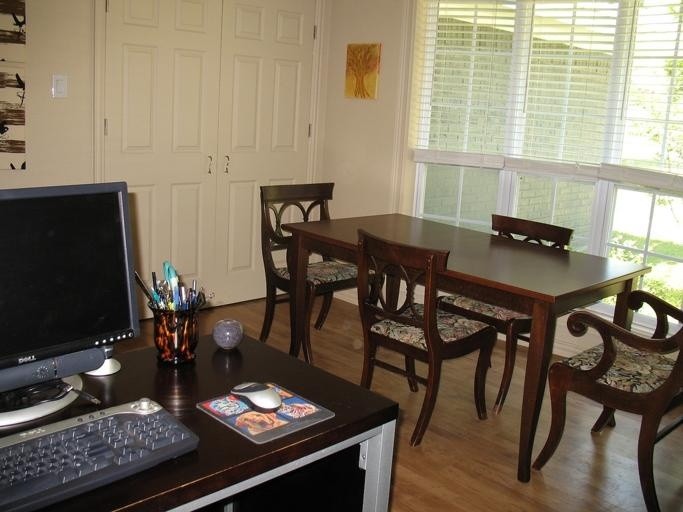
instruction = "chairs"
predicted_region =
[433,215,574,414]
[260,184,385,368]
[532,288,682,512]
[356,227,497,447]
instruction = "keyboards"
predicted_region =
[0,397,200,512]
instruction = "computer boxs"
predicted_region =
[233,460,366,512]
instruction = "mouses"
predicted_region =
[230,381,283,414]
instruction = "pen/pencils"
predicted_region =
[134,260,216,312]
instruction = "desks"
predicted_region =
[282,213,652,485]
[0,332,399,512]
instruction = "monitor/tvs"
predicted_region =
[0,181,141,428]
